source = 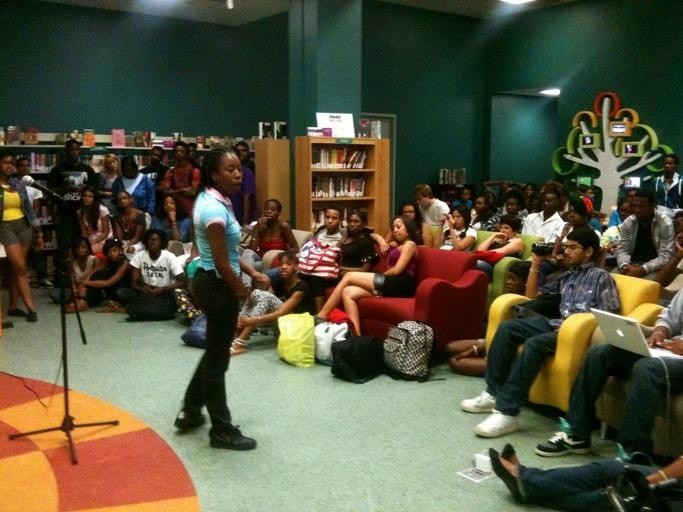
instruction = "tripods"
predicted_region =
[6,209,118,463]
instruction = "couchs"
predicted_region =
[591,372,681,463]
[471,230,545,314]
[325,241,489,357]
[483,270,665,417]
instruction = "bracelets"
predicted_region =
[472,344,478,356]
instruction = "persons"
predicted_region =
[608,200,632,228]
[461,228,621,437]
[415,183,451,247]
[113,190,147,260]
[610,188,675,281]
[538,195,602,288]
[241,199,300,294]
[445,260,532,377]
[162,141,202,216]
[47,139,97,288]
[110,154,155,216]
[65,239,132,313]
[300,205,390,297]
[488,442,683,512]
[48,236,101,304]
[400,202,424,245]
[473,195,495,222]
[0,153,44,322]
[173,146,270,451]
[492,179,515,213]
[523,182,540,214]
[649,153,683,210]
[116,227,187,320]
[534,287,683,457]
[452,187,474,210]
[77,186,114,263]
[100,152,120,216]
[483,191,524,233]
[314,215,419,337]
[139,145,170,192]
[654,210,683,287]
[315,211,378,314]
[433,205,478,252]
[149,192,193,243]
[230,250,310,356]
[521,191,565,243]
[230,146,255,224]
[186,142,201,169]
[476,214,524,283]
[234,141,256,173]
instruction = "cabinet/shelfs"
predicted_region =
[293,135,390,239]
[1,126,63,286]
[67,130,256,176]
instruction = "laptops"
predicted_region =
[590,306,679,358]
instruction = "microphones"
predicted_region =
[22,173,63,203]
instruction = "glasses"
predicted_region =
[561,244,581,250]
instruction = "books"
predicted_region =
[196,135,206,148]
[312,206,351,229]
[439,167,467,184]
[132,130,163,147]
[30,151,175,274]
[4,125,21,145]
[273,121,289,140]
[311,175,367,198]
[234,136,244,144]
[307,118,382,139]
[112,128,126,147]
[311,146,368,170]
[209,135,221,148]
[82,128,96,146]
[173,132,184,142]
[257,121,272,139]
[223,135,234,147]
[24,127,39,144]
[66,129,80,141]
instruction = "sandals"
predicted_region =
[501,443,519,464]
[229,337,247,355]
[489,448,523,503]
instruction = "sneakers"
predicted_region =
[209,426,256,451]
[473,409,520,437]
[461,389,496,414]
[7,308,27,317]
[534,431,593,456]
[174,408,205,429]
[27,311,37,322]
[65,299,88,313]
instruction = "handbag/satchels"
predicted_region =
[315,321,348,365]
[298,239,340,279]
[330,335,382,383]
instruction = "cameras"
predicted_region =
[531,242,551,256]
